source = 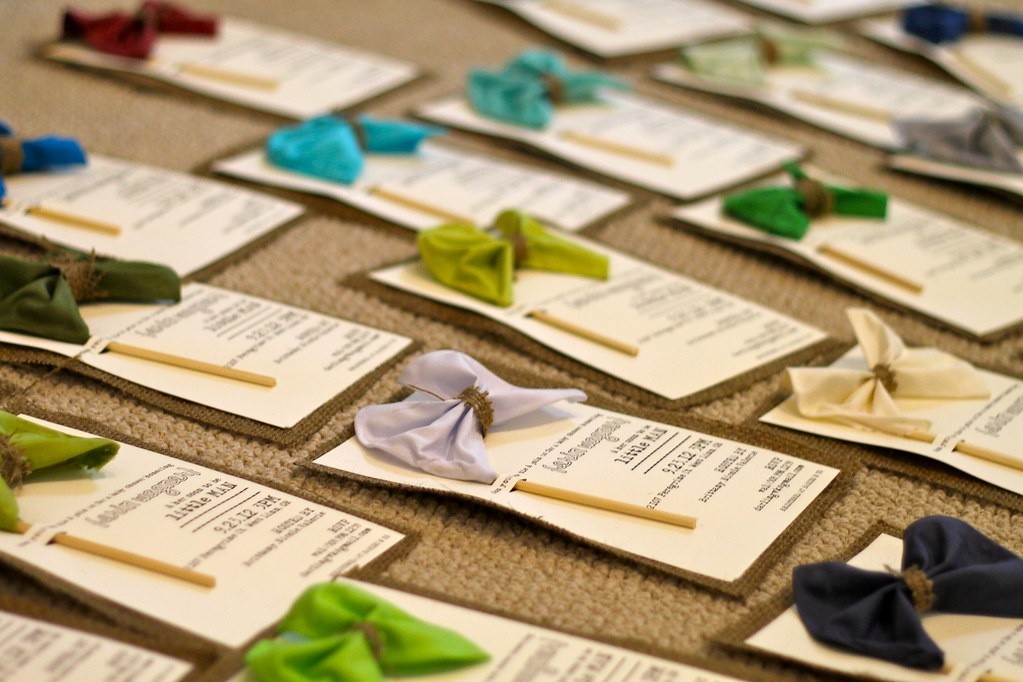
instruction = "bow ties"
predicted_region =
[0,119,89,208]
[780,308,993,439]
[263,110,449,186]
[789,514,1022,670]
[62,0,219,61]
[899,0,1023,45]
[673,21,840,93]
[415,207,611,309]
[718,159,889,242]
[1,247,182,346]
[244,576,490,681]
[0,409,121,534]
[353,349,588,488]
[890,98,1022,175]
[463,48,639,132]
